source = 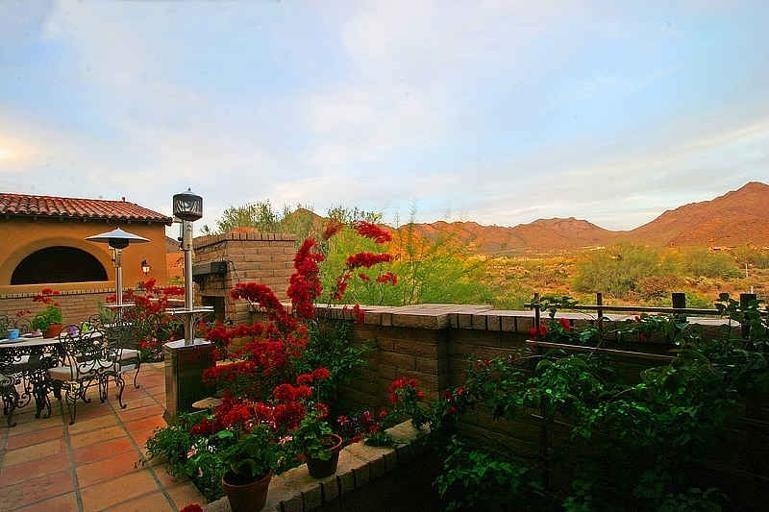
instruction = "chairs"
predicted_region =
[0,315,145,426]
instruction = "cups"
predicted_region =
[80,321,89,331]
[68,325,76,335]
[7,328,19,340]
[31,331,40,335]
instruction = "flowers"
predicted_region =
[22,286,66,333]
[271,368,341,464]
[181,393,285,479]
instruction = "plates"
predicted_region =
[0,339,28,344]
[60,335,78,339]
[22,334,43,337]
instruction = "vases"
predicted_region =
[221,470,271,512]
[299,437,344,479]
